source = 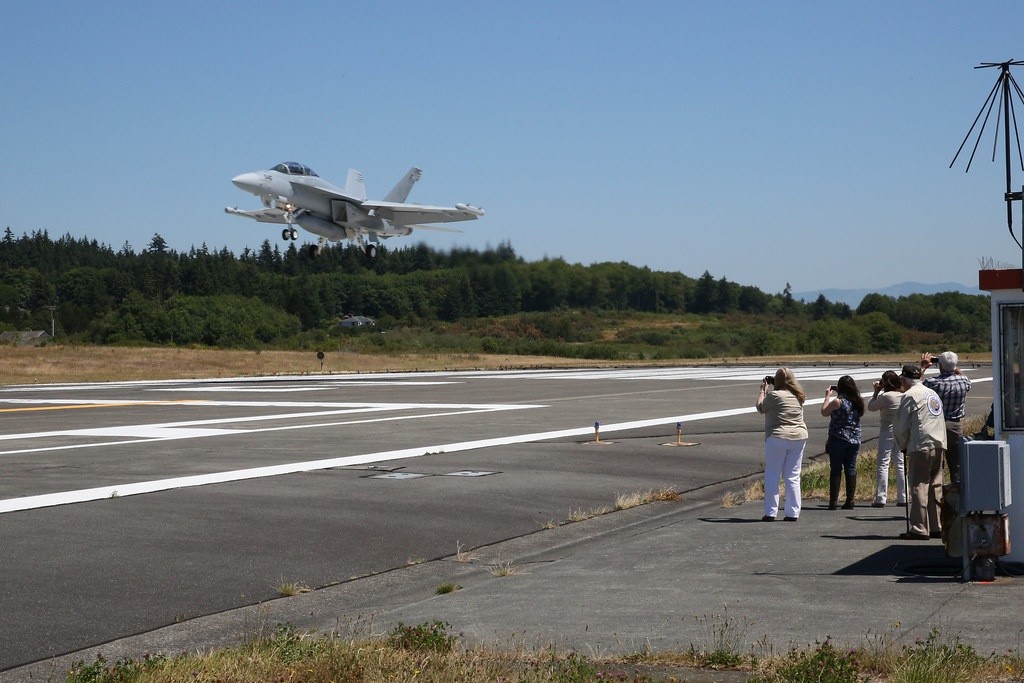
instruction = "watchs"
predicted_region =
[919,368,924,372]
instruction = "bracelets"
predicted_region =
[759,390,765,393]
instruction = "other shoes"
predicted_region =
[900,530,930,540]
[931,531,941,538]
[762,514,774,521]
[872,500,884,507]
[896,502,906,506]
[784,516,797,521]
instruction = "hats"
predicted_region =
[898,365,921,379]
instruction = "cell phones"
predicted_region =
[931,358,939,363]
[831,385,837,390]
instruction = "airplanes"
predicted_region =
[223,163,487,258]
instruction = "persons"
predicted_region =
[821,376,864,510]
[756,368,808,521]
[918,351,972,484]
[868,371,909,508]
[893,365,947,540]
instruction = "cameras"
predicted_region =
[765,376,774,383]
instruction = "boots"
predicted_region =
[828,475,841,510]
[841,475,856,509]
